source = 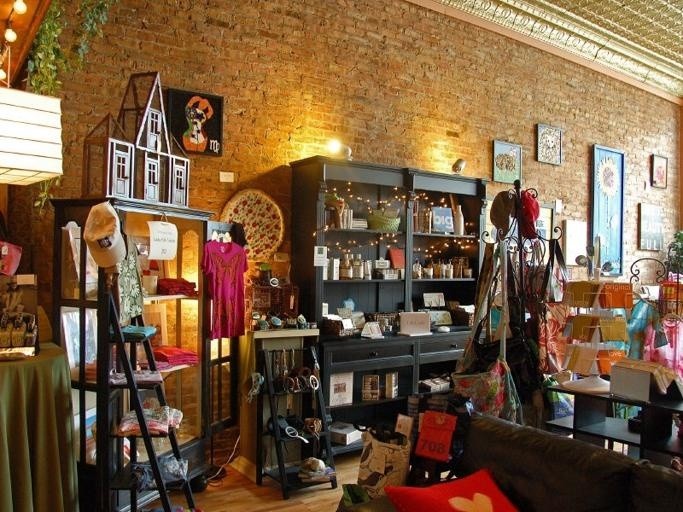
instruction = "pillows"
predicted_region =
[382,468,520,512]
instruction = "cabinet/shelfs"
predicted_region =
[546,384,683,460]
[404,166,488,397]
[289,155,416,458]
[50,196,247,512]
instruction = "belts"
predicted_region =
[267,415,298,439]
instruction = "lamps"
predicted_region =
[5,0,31,45]
[324,137,360,163]
[0,45,64,189]
[453,155,464,172]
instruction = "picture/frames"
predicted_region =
[527,202,558,266]
[535,123,562,167]
[165,88,226,158]
[588,141,626,275]
[650,150,668,190]
[492,140,521,186]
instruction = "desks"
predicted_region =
[1,341,80,512]
[227,325,320,486]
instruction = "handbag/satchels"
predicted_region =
[450,260,571,425]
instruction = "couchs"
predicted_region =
[336,413,683,512]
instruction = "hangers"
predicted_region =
[658,302,683,323]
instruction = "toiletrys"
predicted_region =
[322,253,471,279]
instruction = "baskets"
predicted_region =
[325,319,352,337]
[367,215,401,232]
[452,309,474,326]
[367,312,398,329]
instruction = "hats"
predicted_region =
[83,201,126,269]
[489,190,511,242]
[517,191,539,238]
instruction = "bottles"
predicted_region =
[453,204,464,236]
[413,256,455,280]
[340,252,364,281]
